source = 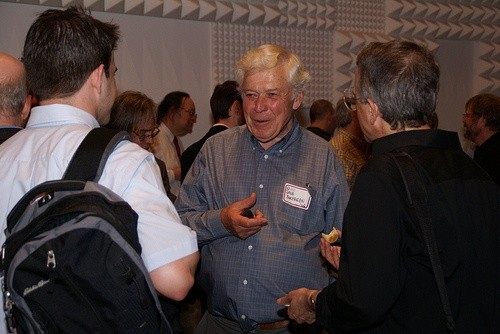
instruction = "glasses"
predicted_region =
[343,88,367,112]
[133,127,160,141]
[179,109,197,119]
[463,114,481,119]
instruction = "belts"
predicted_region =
[260,320,289,330]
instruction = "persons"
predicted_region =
[174,44,350,334]
[0,8,200,334]
[180,81,245,185]
[306,100,336,142]
[463,93,500,187]
[150,92,196,198]
[102,90,176,205]
[277,41,500,334]
[0,52,32,145]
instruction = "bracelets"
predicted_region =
[310,290,320,310]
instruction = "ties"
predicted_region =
[173,136,181,163]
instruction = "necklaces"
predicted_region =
[329,98,370,191]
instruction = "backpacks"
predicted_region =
[0,128,173,334]
